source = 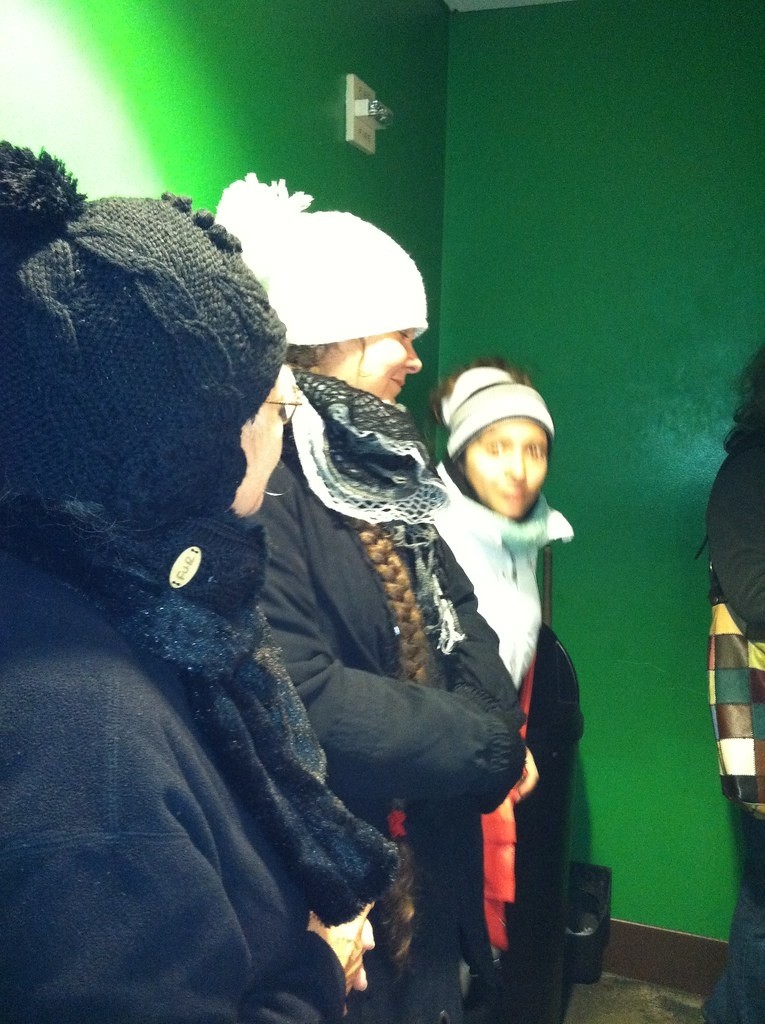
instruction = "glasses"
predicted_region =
[263,382,303,423]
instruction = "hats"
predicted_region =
[438,366,555,462]
[0,140,286,533]
[215,172,429,344]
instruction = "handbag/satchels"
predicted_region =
[708,588,765,820]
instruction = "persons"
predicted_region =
[1,143,585,1024]
[699,337,765,1024]
